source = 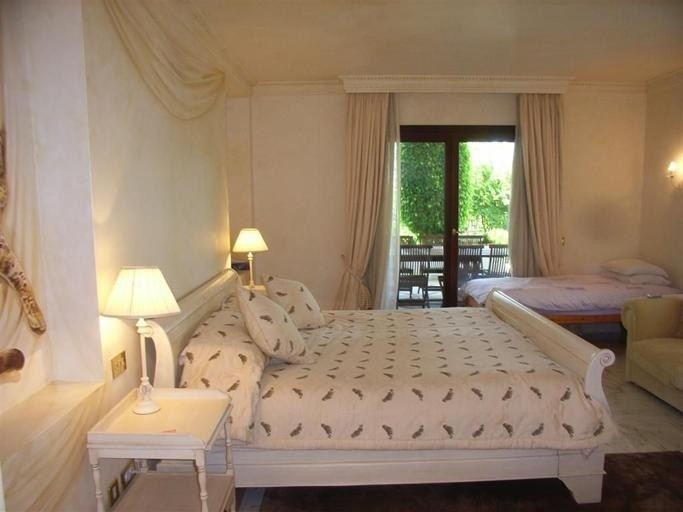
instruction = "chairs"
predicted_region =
[398,230,513,307]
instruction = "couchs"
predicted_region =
[619,291,683,413]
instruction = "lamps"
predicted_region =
[665,159,682,193]
[231,225,268,290]
[95,260,183,415]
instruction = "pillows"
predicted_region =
[614,271,672,285]
[259,273,327,330]
[597,255,672,278]
[234,285,314,369]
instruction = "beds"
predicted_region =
[138,269,628,506]
[459,272,682,327]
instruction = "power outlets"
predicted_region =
[107,349,130,381]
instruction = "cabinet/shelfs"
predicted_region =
[82,380,237,511]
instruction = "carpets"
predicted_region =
[255,447,681,511]
